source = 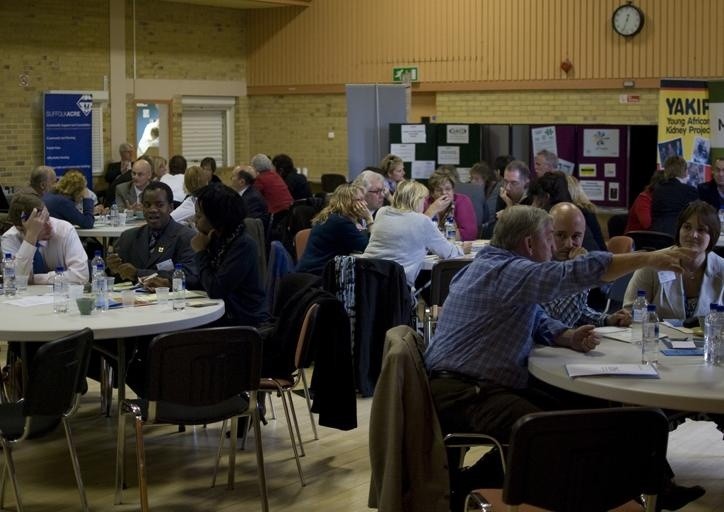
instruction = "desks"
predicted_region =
[423,239,489,270]
[0,284,225,409]
[73,215,147,258]
[529,320,724,414]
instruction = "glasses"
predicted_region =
[368,189,385,194]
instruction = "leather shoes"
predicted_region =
[656,486,705,512]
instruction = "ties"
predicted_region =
[149,232,156,254]
[33,242,44,273]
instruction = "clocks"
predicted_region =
[612,4,645,38]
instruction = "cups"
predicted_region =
[121,290,135,310]
[105,277,115,292]
[155,288,170,307]
[77,297,95,315]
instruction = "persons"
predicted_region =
[143,182,269,437]
[1,192,90,440]
[0,141,724,329]
[421,203,708,511]
[104,181,202,424]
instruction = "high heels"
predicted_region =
[226,402,267,437]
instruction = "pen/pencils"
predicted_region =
[20,209,40,219]
[135,273,159,287]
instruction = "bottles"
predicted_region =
[445,217,451,229]
[93,264,108,309]
[53,267,68,313]
[172,263,187,311]
[446,220,456,243]
[709,305,724,367]
[111,202,119,227]
[642,304,661,366]
[3,253,16,296]
[704,302,717,362]
[632,290,649,341]
[91,250,104,280]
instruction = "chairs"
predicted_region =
[608,214,626,239]
[455,183,485,240]
[113,325,269,512]
[464,408,669,512]
[624,230,675,251]
[0,328,94,512]
[211,271,357,487]
[601,236,634,314]
[368,324,510,512]
[430,259,474,305]
[244,173,346,310]
